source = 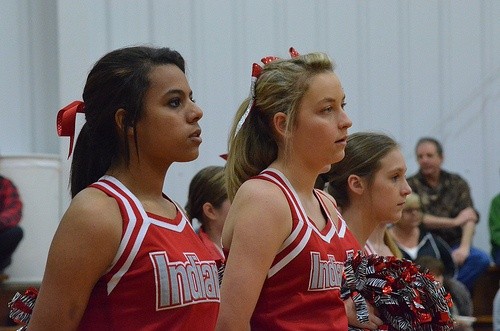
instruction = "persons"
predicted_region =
[222,52,348,331]
[28,46,222,331]
[1,175,24,281]
[313,131,454,330]
[407,137,490,296]
[184,165,230,263]
[415,256,459,316]
[487,193,500,331]
[387,182,473,315]
